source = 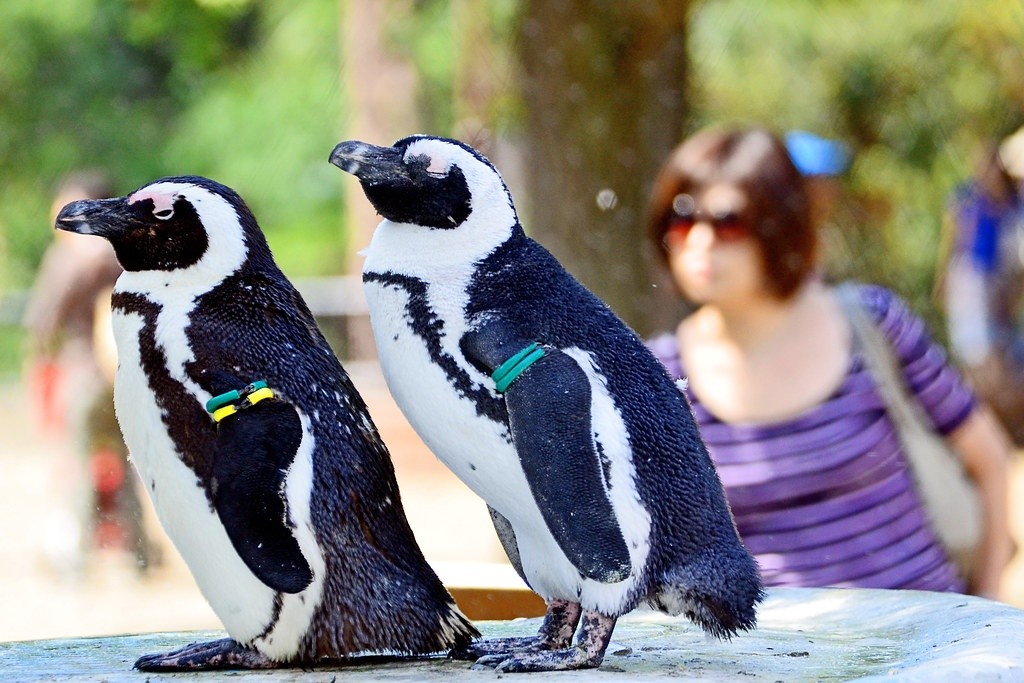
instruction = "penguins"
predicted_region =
[54,175,482,672]
[327,136,769,671]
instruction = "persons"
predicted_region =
[645,112,1024,607]
[25,172,148,555]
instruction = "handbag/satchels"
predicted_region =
[834,280,981,551]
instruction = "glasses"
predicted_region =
[658,205,756,249]
